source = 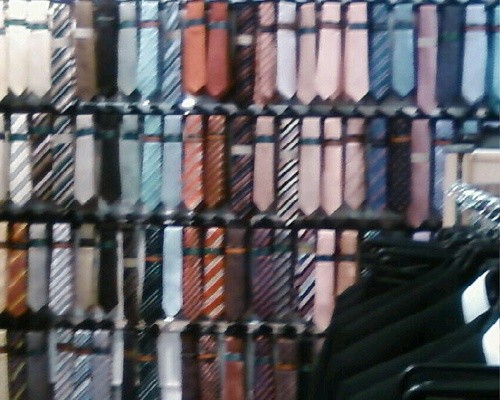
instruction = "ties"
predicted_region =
[8,111,33,209]
[252,2,277,108]
[141,223,165,326]
[76,115,95,206]
[139,114,164,212]
[385,115,412,216]
[220,332,247,399]
[91,328,113,399]
[291,227,318,326]
[116,2,140,98]
[435,3,463,111]
[181,226,203,324]
[159,2,183,110]
[310,228,337,338]
[72,329,93,400]
[179,327,202,399]
[252,330,278,400]
[119,324,139,400]
[99,112,122,204]
[94,0,118,99]
[183,2,207,95]
[461,2,488,107]
[251,114,276,216]
[196,330,222,400]
[160,225,185,320]
[276,116,301,229]
[47,221,74,319]
[135,1,161,102]
[233,4,257,110]
[404,118,432,227]
[228,114,254,222]
[484,117,499,147]
[51,324,76,399]
[336,225,361,297]
[315,2,343,102]
[415,3,439,118]
[390,2,417,98]
[249,225,275,324]
[160,113,183,212]
[203,113,227,210]
[25,221,49,316]
[343,2,370,104]
[295,2,318,107]
[7,327,29,400]
[6,221,29,318]
[319,116,344,217]
[0,329,11,400]
[271,226,294,322]
[76,222,96,312]
[0,220,8,318]
[342,115,367,212]
[97,222,119,314]
[121,221,140,329]
[201,227,226,323]
[275,1,298,102]
[181,112,206,212]
[462,117,483,147]
[491,5,500,114]
[205,2,231,97]
[368,2,392,105]
[0,110,7,208]
[24,328,51,400]
[137,324,161,400]
[297,115,323,217]
[276,335,300,399]
[364,116,389,219]
[31,110,53,215]
[0,1,78,114]
[52,115,76,217]
[118,111,142,210]
[222,225,249,323]
[432,115,456,217]
[73,1,97,100]
